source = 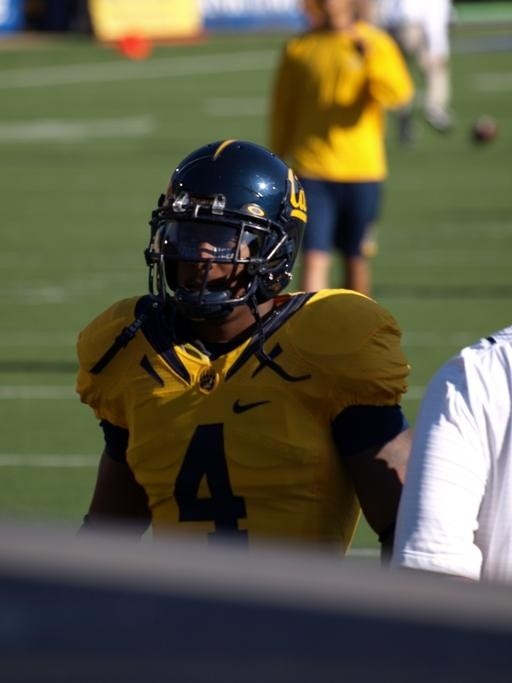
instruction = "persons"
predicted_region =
[388,325,512,588]
[76,140,412,570]
[383,2,456,140]
[269,0,414,303]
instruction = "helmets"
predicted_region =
[154,135,311,302]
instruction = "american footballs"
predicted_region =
[468,116,496,143]
